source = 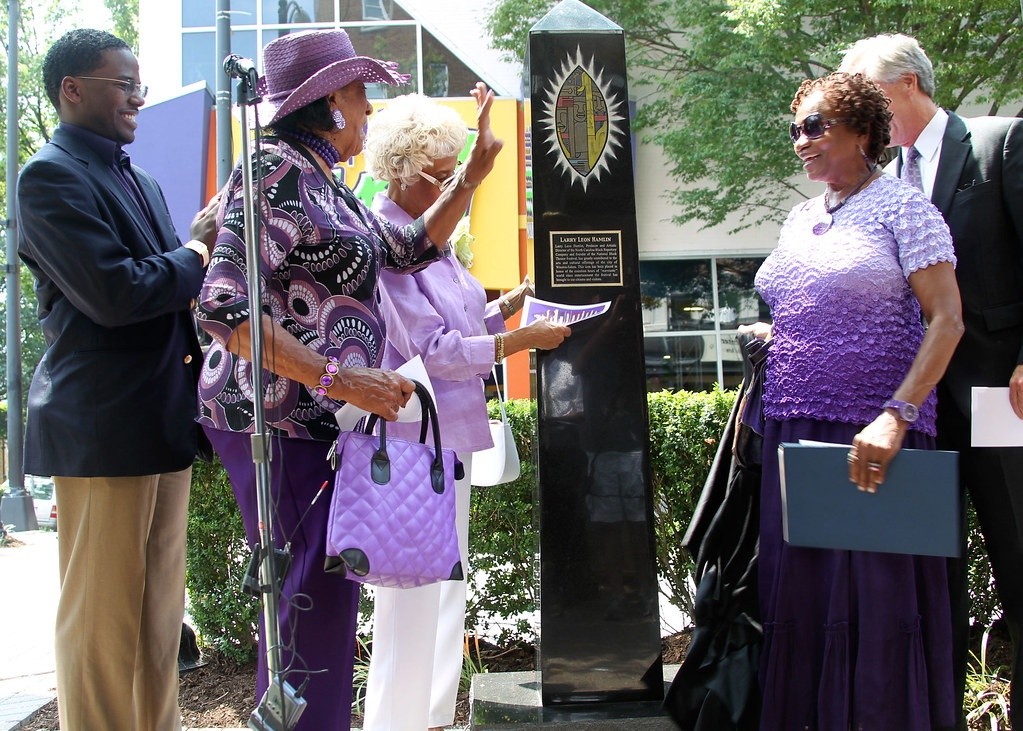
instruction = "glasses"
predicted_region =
[790,114,858,144]
[75,74,148,99]
[414,170,461,193]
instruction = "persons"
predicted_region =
[747,70,968,731]
[15,29,229,731]
[194,27,506,731]
[836,32,1022,731]
[355,96,571,731]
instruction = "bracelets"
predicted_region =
[500,296,515,316]
[499,332,504,364]
[883,399,921,424]
[456,170,481,190]
[313,356,340,397]
[494,334,501,364]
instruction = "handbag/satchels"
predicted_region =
[324,376,463,589]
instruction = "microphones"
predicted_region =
[223,54,255,79]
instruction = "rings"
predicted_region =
[868,466,880,472]
[845,459,855,464]
[846,451,860,460]
[867,461,883,467]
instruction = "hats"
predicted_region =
[237,28,412,129]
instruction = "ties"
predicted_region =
[902,145,925,197]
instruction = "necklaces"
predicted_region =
[287,129,340,170]
[811,166,877,235]
